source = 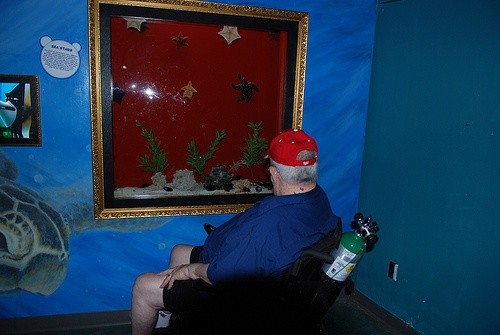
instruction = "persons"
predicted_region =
[129,128,337,335]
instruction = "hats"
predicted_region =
[263,129,318,166]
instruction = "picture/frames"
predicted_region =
[87,0,310,220]
[0,73,42,146]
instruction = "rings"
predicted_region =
[168,274,170,277]
